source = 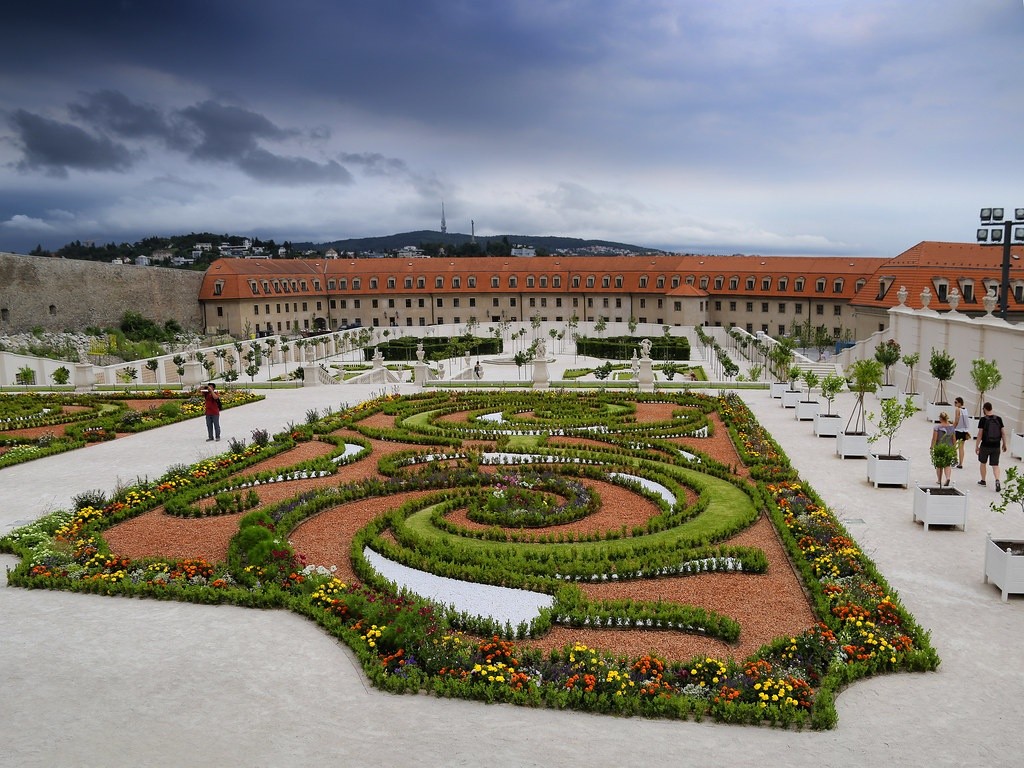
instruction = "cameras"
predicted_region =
[204,386,208,389]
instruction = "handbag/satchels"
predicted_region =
[965,432,971,440]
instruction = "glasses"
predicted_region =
[955,401,957,404]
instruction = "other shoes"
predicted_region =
[950,461,958,467]
[956,465,962,468]
[936,482,940,484]
[206,437,214,441]
[944,479,950,486]
[215,437,220,441]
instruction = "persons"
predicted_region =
[975,402,1007,492]
[250,332,256,339]
[196,383,220,442]
[953,397,968,468]
[820,352,826,363]
[474,361,481,377]
[689,370,696,381]
[930,412,956,486]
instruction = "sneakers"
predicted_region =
[977,480,986,486]
[995,479,1001,492]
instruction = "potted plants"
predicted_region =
[867,395,922,488]
[899,352,923,410]
[837,358,885,460]
[968,357,1002,440]
[925,347,956,423]
[780,365,803,408]
[873,338,901,399]
[913,432,969,532]
[768,332,799,398]
[795,368,820,420]
[813,372,846,437]
[789,365,801,391]
[984,466,1024,601]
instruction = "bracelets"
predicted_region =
[930,447,933,449]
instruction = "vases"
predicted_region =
[1010,429,1024,462]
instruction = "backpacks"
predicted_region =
[215,396,222,411]
[984,415,1001,442]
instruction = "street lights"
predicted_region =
[974,205,1024,320]
